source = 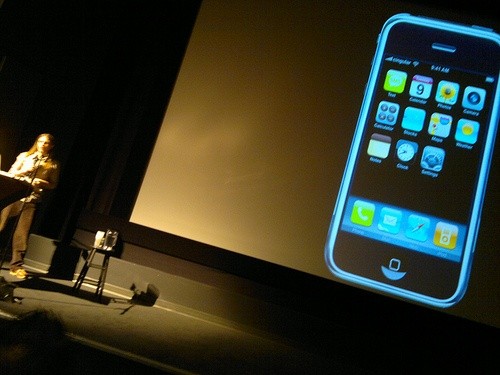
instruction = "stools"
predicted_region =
[72,245,115,302]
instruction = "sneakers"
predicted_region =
[9,266,32,278]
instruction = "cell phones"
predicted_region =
[325,13,500,307]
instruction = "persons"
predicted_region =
[0,132,60,279]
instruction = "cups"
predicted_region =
[103,229,119,251]
[94,230,106,248]
